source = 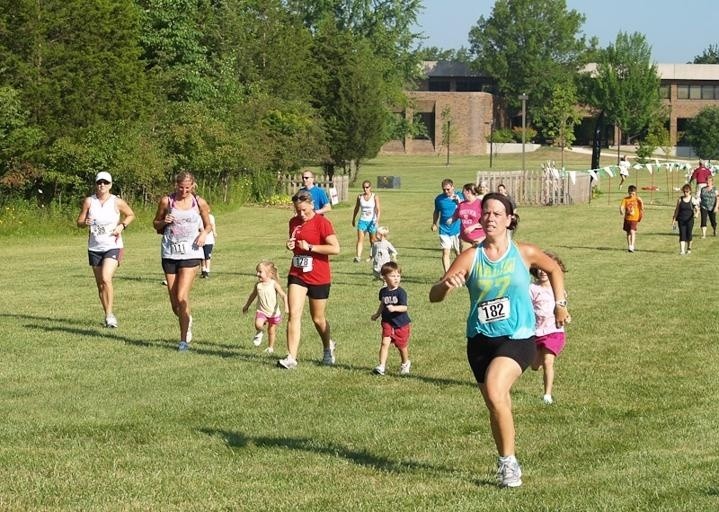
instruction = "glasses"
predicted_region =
[97,180,109,185]
[292,195,308,202]
[443,188,450,191]
[363,186,369,188]
[301,176,309,179]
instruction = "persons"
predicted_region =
[77,172,135,328]
[497,184,518,239]
[299,171,331,217]
[242,261,289,352]
[618,156,631,189]
[476,181,490,198]
[367,225,399,288]
[371,262,412,376]
[672,185,699,254]
[352,181,381,263]
[279,191,341,368]
[689,159,713,219]
[432,179,464,273]
[430,192,571,487]
[200,204,218,278]
[152,172,211,349]
[619,185,645,252]
[446,183,486,254]
[700,176,719,238]
[163,280,168,285]
[529,252,568,404]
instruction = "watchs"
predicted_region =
[556,298,568,307]
[309,244,312,252]
[120,223,126,230]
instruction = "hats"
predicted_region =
[95,171,113,182]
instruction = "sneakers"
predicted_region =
[200,271,204,278]
[544,395,552,404]
[322,340,336,365]
[162,280,168,285]
[686,248,691,253]
[105,315,117,328]
[204,272,208,278]
[400,359,411,376]
[278,356,298,369]
[252,331,263,346]
[628,246,634,252]
[353,257,360,263]
[186,316,193,343]
[496,458,503,485]
[700,235,705,239]
[500,456,522,488]
[177,341,187,352]
[265,347,273,353]
[679,250,685,256]
[372,365,386,376]
